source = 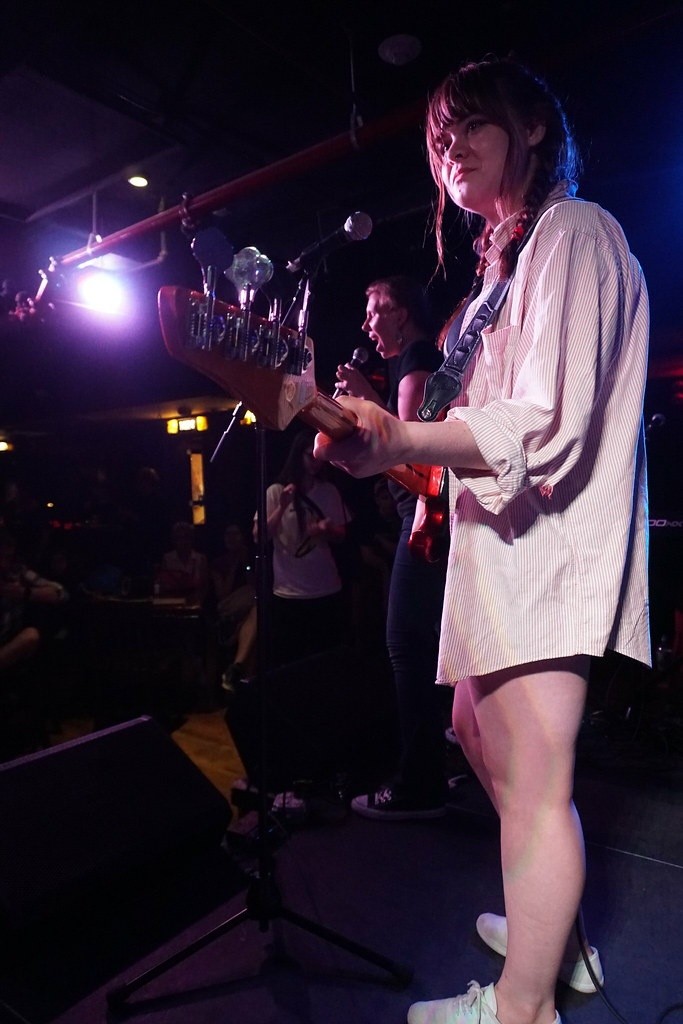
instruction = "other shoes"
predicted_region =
[294,777,326,798]
[324,769,358,803]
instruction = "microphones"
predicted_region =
[333,345,368,400]
[286,211,373,274]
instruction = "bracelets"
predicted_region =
[23,587,31,601]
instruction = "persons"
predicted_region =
[308,61,654,1024]
[330,273,450,819]
[604,373,683,686]
[0,439,359,801]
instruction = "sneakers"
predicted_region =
[406,980,561,1024]
[476,913,605,994]
[445,728,460,744]
[350,779,448,818]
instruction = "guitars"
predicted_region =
[156,223,450,567]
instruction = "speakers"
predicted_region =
[0,716,232,1024]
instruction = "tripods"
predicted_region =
[105,271,415,1013]
